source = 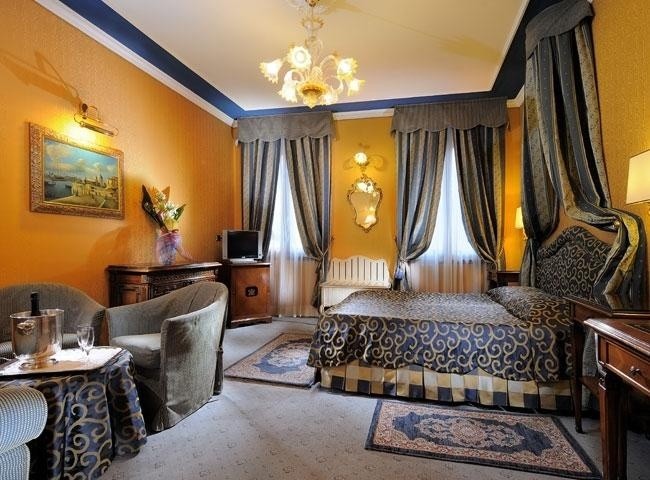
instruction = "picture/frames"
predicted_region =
[29,122,125,220]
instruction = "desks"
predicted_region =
[495,271,520,287]
[563,294,650,480]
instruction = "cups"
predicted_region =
[76,327,85,363]
[81,327,95,362]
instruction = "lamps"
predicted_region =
[625,149,650,216]
[343,152,385,233]
[260,1,367,110]
[514,206,528,241]
[72,102,119,137]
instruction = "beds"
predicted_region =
[306,202,647,422]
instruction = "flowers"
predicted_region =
[140,184,187,268]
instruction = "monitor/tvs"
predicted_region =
[222,230,263,263]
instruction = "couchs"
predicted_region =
[104,280,229,433]
[1,281,106,366]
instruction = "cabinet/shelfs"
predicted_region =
[105,260,223,307]
[223,260,272,328]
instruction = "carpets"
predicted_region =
[223,332,322,389]
[363,397,603,480]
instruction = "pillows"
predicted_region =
[487,285,570,323]
